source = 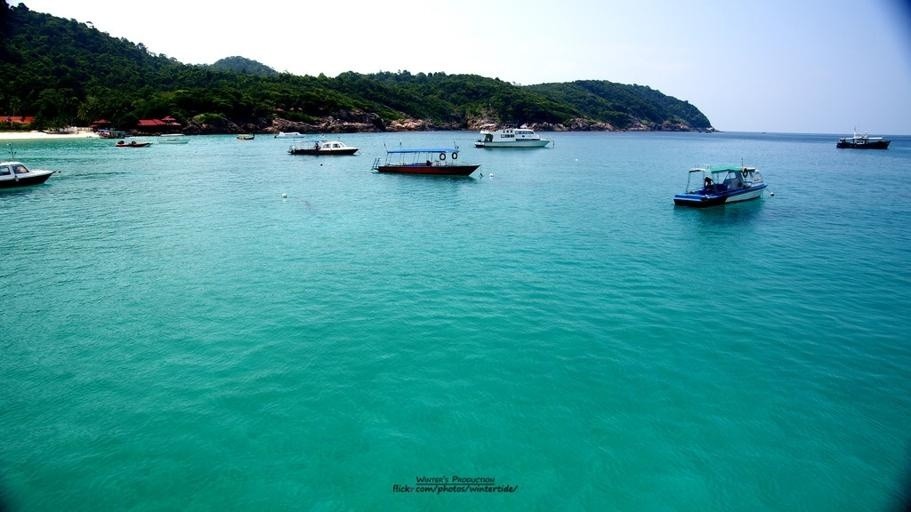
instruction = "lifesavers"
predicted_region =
[439,153,447,161]
[452,152,457,158]
[741,167,748,178]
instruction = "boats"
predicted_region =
[116,139,155,148]
[274,130,306,140]
[674,159,771,207]
[288,141,360,156]
[158,132,192,145]
[1,144,59,189]
[374,145,483,176]
[237,133,255,140]
[474,127,552,149]
[836,128,893,152]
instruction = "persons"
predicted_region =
[704,176,715,188]
[314,143,320,149]
